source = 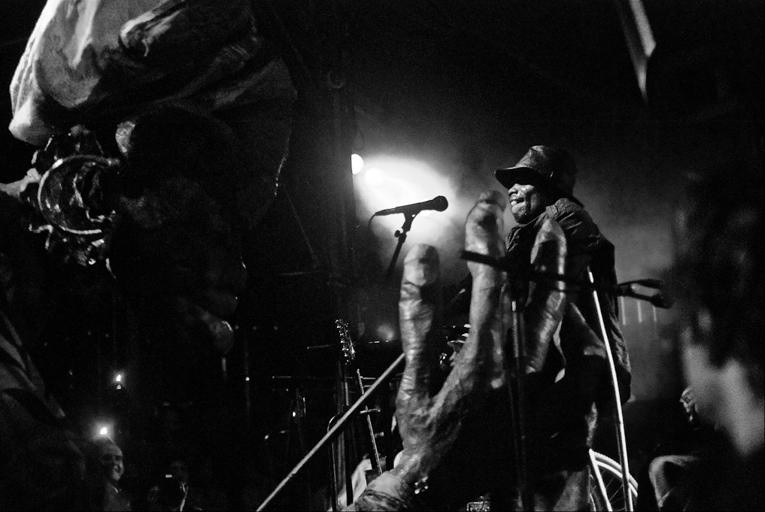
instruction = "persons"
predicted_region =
[341,189,605,512]
[645,191,765,512]
[494,145,631,512]
[93,437,203,512]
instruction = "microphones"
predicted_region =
[637,278,688,296]
[375,196,448,216]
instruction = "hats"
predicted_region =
[494,146,576,190]
